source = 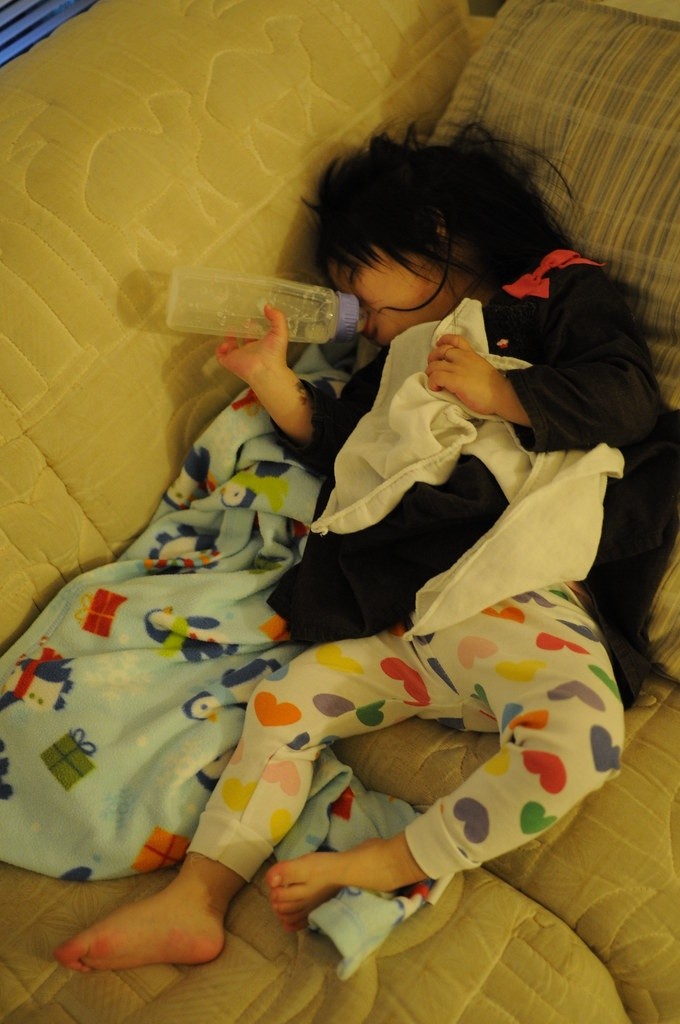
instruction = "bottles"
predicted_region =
[165,262,368,345]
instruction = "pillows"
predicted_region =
[426,0,680,412]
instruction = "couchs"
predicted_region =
[0,0,680,1024]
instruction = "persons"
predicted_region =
[52,118,660,971]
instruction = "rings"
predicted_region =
[441,345,454,360]
